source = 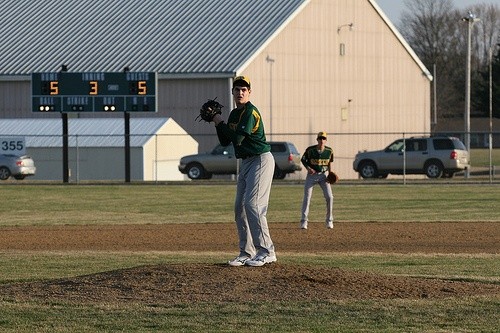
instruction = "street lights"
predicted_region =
[461,10,481,179]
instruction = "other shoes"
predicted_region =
[301,221,308,229]
[327,220,333,229]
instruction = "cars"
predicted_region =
[0,152,37,180]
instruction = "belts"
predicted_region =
[242,153,254,160]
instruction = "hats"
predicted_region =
[233,76,250,85]
[317,132,327,140]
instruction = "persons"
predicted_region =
[300,132,338,229]
[199,76,277,266]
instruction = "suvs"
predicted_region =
[353,136,470,179]
[178,138,302,181]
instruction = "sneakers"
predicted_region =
[230,256,253,266]
[245,254,277,266]
